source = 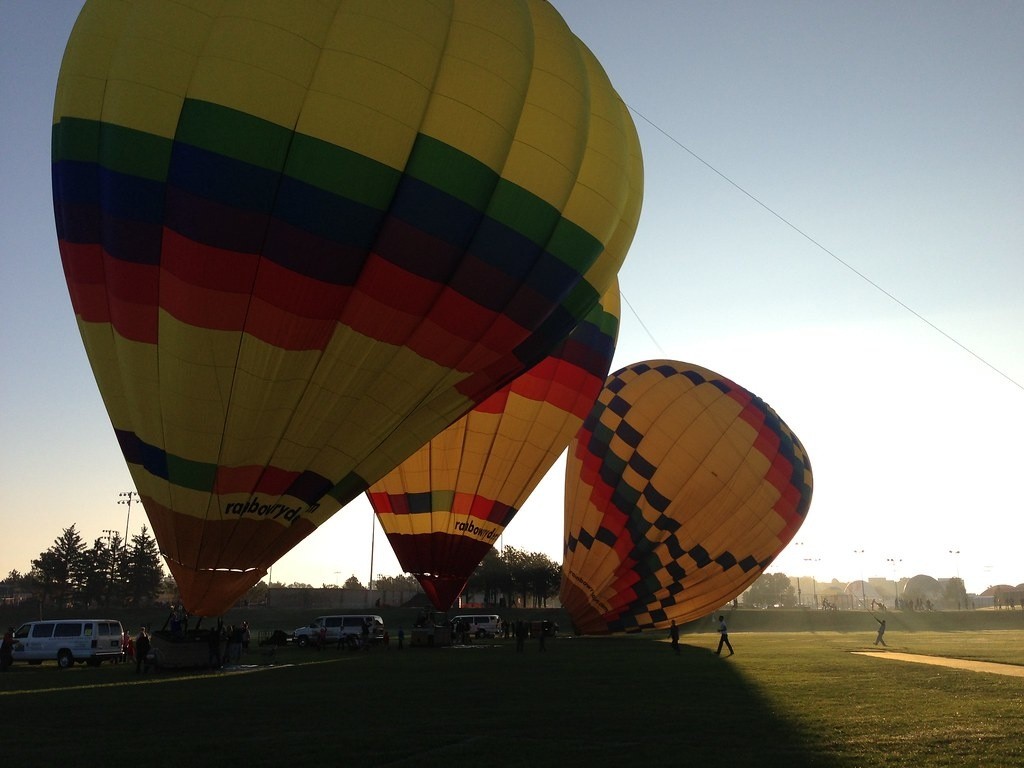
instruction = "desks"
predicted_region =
[367,636,384,648]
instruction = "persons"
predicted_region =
[872,615,887,646]
[169,602,189,639]
[206,617,251,667]
[318,626,328,651]
[109,627,152,675]
[502,617,530,654]
[382,632,390,650]
[412,607,435,648]
[667,619,679,644]
[397,626,404,651]
[450,620,480,645]
[538,631,546,654]
[714,616,734,655]
[0,626,20,671]
[336,626,348,651]
[361,621,383,651]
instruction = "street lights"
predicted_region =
[804,558,821,596]
[887,558,902,599]
[854,549,866,600]
[948,551,960,577]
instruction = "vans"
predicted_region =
[292,614,385,649]
[0,618,126,669]
[449,615,502,640]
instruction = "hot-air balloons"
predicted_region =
[366,272,621,648]
[49,1,644,678]
[529,360,814,642]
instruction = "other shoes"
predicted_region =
[714,652,720,656]
[873,643,877,646]
[728,653,734,656]
[884,645,886,646]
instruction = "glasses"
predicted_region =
[12,631,15,632]
